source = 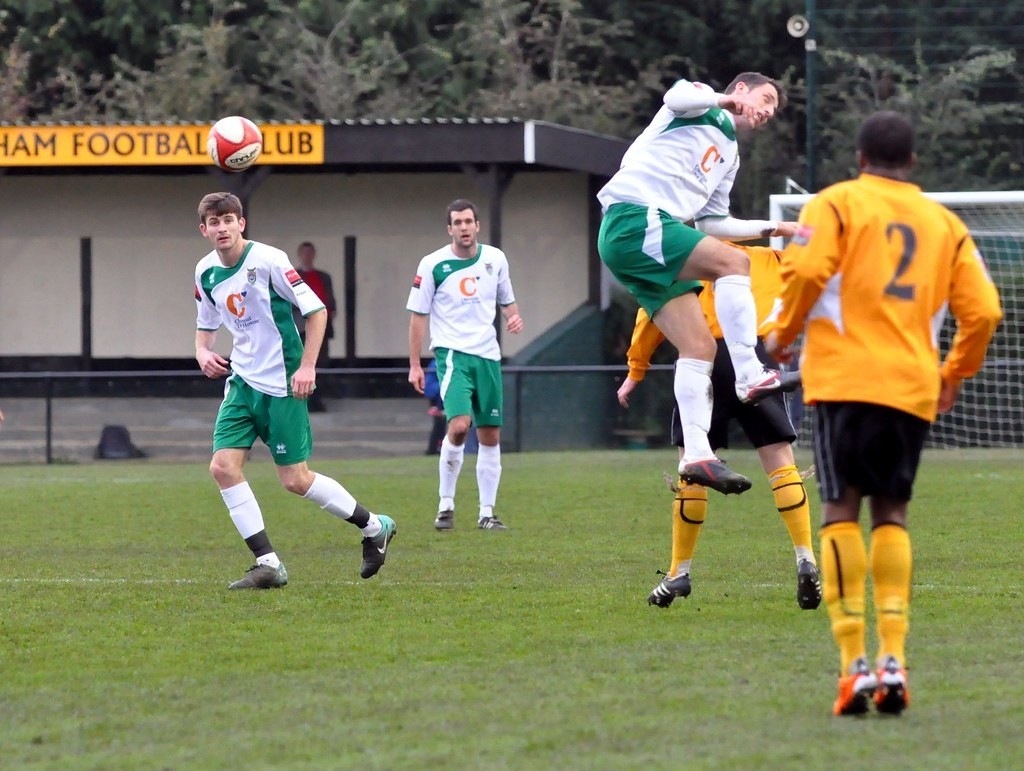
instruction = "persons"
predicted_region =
[193,193,395,590]
[617,231,826,611]
[287,242,336,413]
[598,71,803,495]
[403,200,522,530]
[757,114,1004,716]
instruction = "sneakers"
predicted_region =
[478,515,507,530]
[831,658,876,716]
[677,453,752,496]
[735,366,802,407]
[434,509,455,531]
[647,572,692,609]
[795,559,822,610]
[873,655,909,715]
[228,562,287,590]
[361,514,396,579]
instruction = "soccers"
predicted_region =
[205,116,265,174]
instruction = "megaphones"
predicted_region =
[786,15,811,38]
